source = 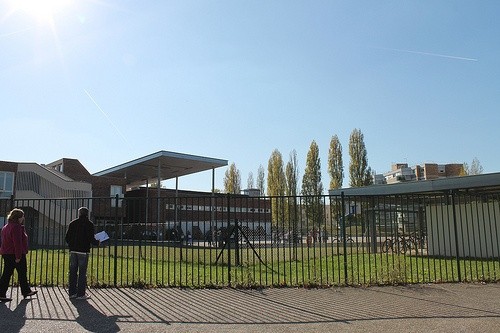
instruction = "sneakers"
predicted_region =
[69,293,77,299]
[76,295,91,299]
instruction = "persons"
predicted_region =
[0,208,38,301]
[170,229,192,246]
[207,225,234,247]
[284,226,329,246]
[65,207,101,299]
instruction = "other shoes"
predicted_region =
[0,299,12,301]
[22,291,38,296]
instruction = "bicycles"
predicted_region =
[332,234,354,243]
[382,229,425,254]
